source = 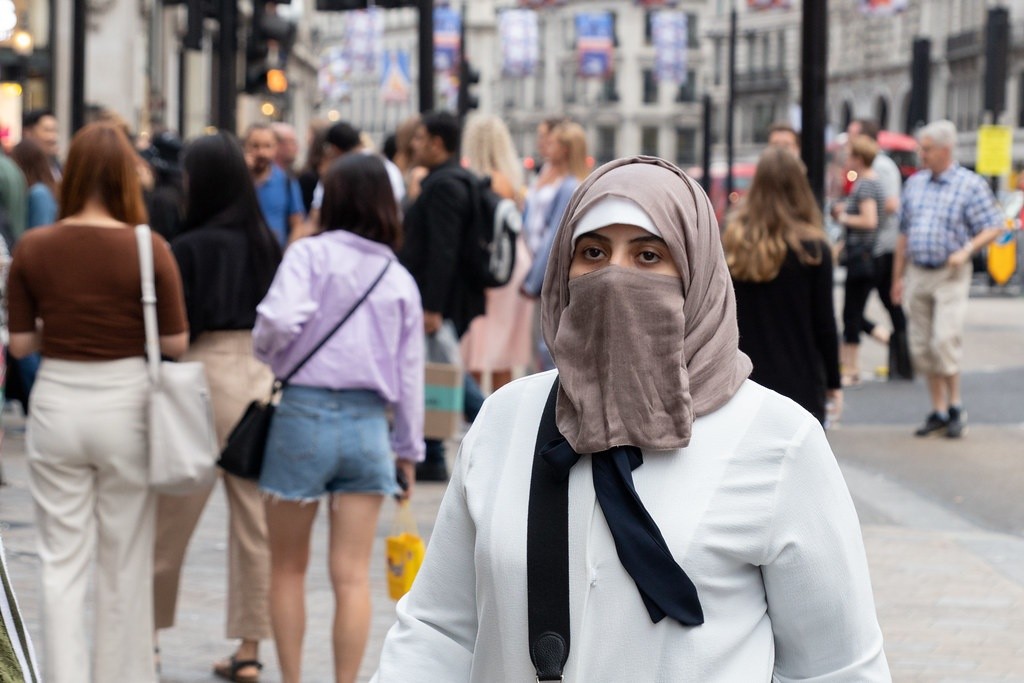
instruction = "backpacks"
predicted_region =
[427,164,523,288]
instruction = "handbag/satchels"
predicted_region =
[838,241,872,266]
[425,320,464,439]
[386,495,425,600]
[148,361,220,500]
[216,399,276,481]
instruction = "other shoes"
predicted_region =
[914,412,950,437]
[840,365,859,385]
[414,460,448,483]
[947,404,966,439]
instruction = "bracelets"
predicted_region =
[963,241,977,258]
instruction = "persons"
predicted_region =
[252,153,426,683]
[1,107,1024,484]
[363,153,897,683]
[153,131,272,683]
[5,119,191,683]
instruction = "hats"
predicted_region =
[140,132,190,174]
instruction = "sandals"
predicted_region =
[214,653,262,683]
